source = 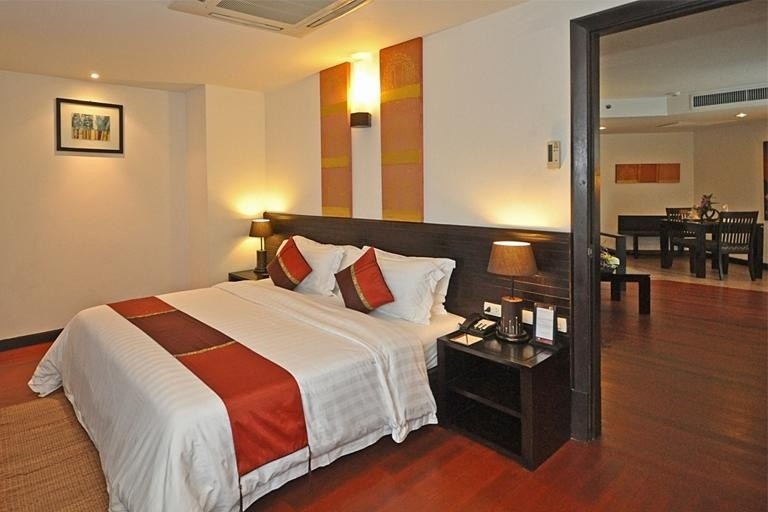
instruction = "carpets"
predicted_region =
[0,389,110,512]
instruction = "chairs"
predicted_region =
[660,206,764,281]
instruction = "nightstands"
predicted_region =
[228,270,260,280]
[436,327,569,472]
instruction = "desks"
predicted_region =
[601,266,650,314]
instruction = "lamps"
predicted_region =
[249,219,272,273]
[487,240,538,344]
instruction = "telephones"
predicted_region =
[460,312,500,337]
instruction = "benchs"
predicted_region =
[617,214,662,257]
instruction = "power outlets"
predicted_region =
[484,302,501,318]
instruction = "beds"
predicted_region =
[27,277,466,512]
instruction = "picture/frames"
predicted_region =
[56,98,123,154]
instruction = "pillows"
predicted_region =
[334,248,394,315]
[362,245,456,316]
[329,243,361,297]
[267,237,311,290]
[276,235,344,298]
[337,261,444,326]
